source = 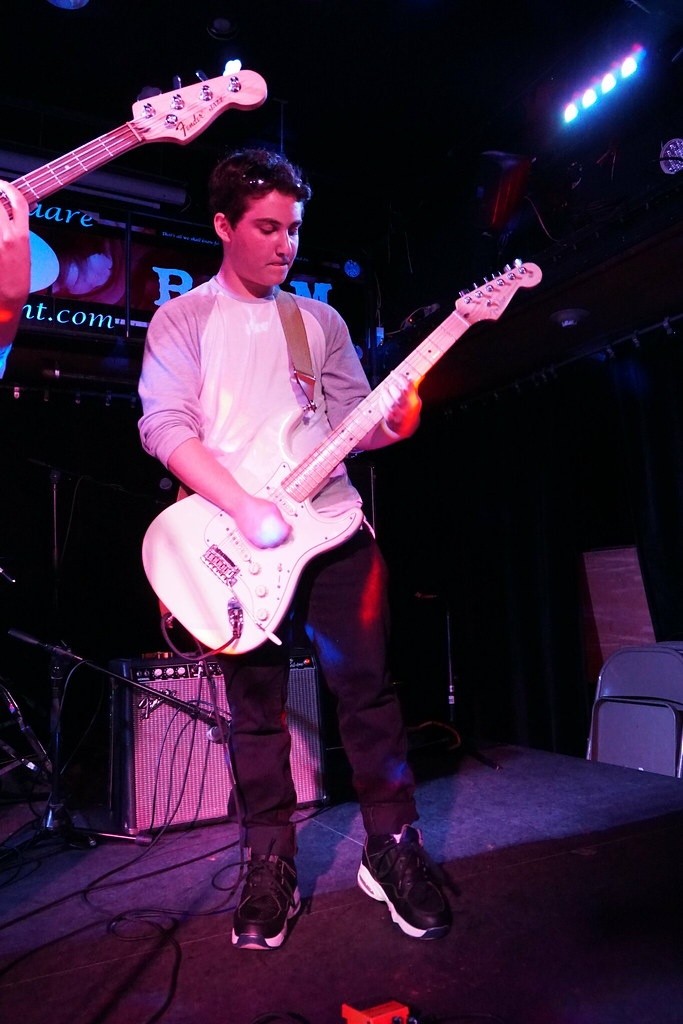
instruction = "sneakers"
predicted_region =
[356,825,450,940]
[231,856,299,950]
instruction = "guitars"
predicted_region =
[136,253,549,666]
[1,54,271,224]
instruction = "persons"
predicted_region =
[0,175,29,381]
[138,149,453,952]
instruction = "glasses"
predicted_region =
[235,165,303,193]
